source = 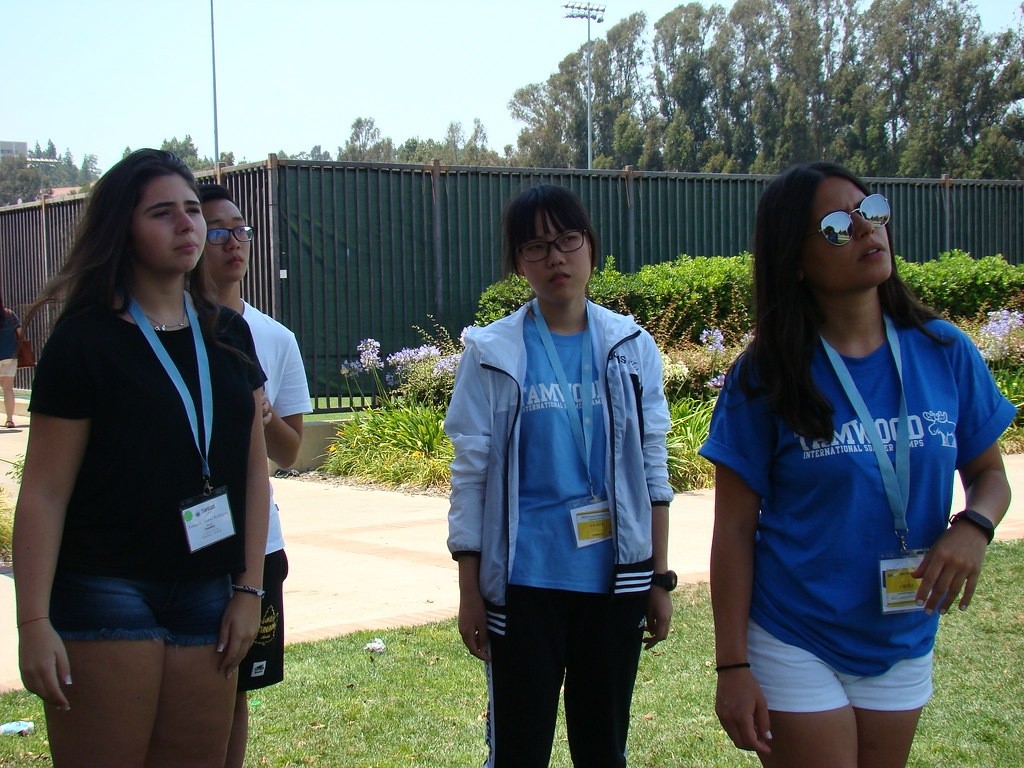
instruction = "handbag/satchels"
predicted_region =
[4,308,36,368]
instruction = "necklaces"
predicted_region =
[146,308,186,332]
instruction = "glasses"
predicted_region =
[805,193,890,246]
[518,229,587,263]
[206,225,255,245]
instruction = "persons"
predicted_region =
[1,294,27,427]
[184,184,315,767]
[699,162,1019,768]
[12,148,270,768]
[442,183,679,768]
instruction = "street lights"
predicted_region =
[562,2,608,167]
[26,156,62,338]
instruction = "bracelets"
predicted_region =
[715,662,751,672]
[230,584,266,599]
[17,615,50,630]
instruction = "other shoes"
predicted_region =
[4,421,15,428]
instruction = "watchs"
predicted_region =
[651,570,677,591]
[949,507,995,547]
